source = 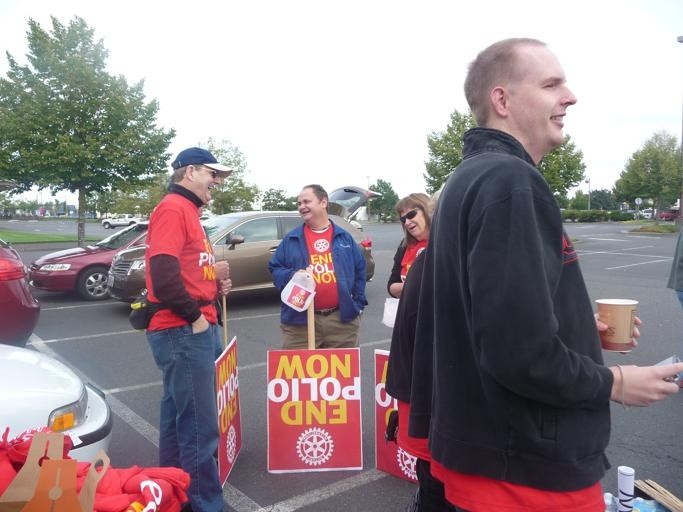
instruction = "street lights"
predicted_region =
[585,177,590,210]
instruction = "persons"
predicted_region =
[383,248,459,511]
[146,147,234,509]
[614,362,626,409]
[386,192,434,298]
[425,38,681,510]
[266,183,368,347]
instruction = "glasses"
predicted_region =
[195,166,218,178]
[399,206,423,223]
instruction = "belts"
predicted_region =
[313,306,341,316]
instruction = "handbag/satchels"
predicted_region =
[384,409,400,443]
[128,295,158,330]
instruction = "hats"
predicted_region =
[172,148,232,179]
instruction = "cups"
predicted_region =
[594,298,639,354]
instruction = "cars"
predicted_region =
[0,341,113,465]
[642,208,679,221]
[0,236,40,348]
